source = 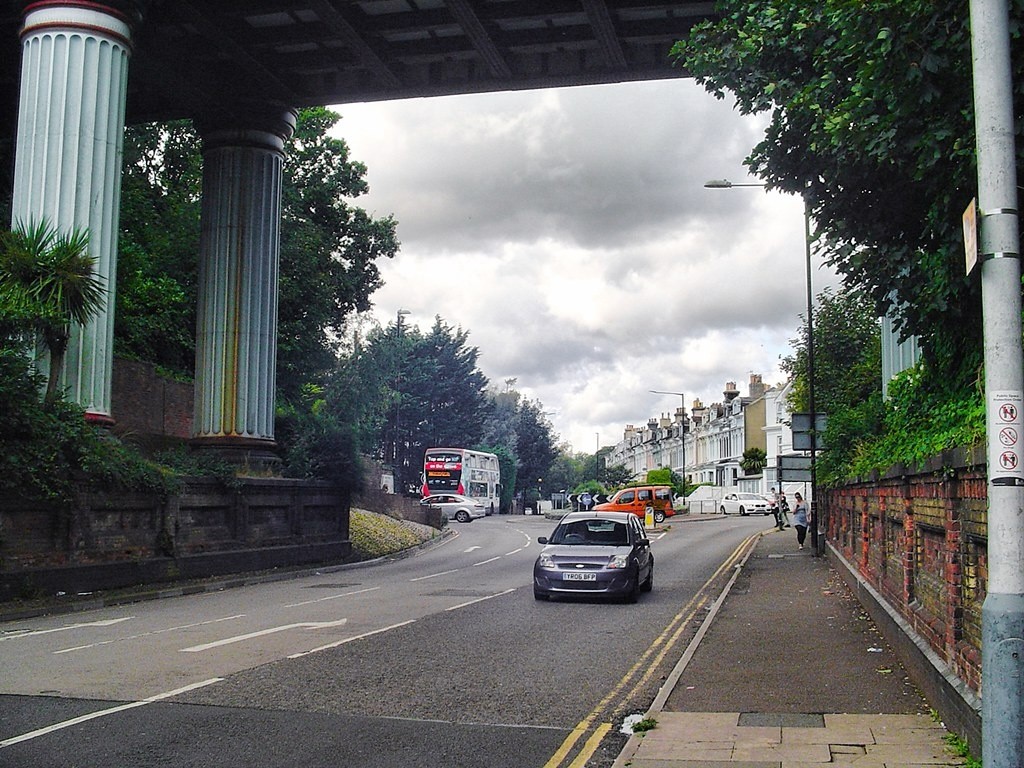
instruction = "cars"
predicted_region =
[720,492,773,517]
[525,508,533,515]
[420,494,483,522]
[533,511,655,604]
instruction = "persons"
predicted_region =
[778,491,791,527]
[792,492,810,550]
[769,487,782,527]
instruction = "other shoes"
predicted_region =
[799,544,803,550]
[784,524,791,527]
[774,522,779,527]
[782,521,785,526]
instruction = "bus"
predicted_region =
[423,447,503,516]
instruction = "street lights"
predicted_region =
[596,432,599,483]
[703,179,819,557]
[395,309,411,464]
[649,390,686,506]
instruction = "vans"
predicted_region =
[591,486,674,523]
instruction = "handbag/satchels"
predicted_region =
[805,503,811,523]
[783,503,790,512]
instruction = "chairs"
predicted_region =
[571,522,588,538]
[613,524,629,542]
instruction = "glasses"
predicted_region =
[796,496,800,499]
